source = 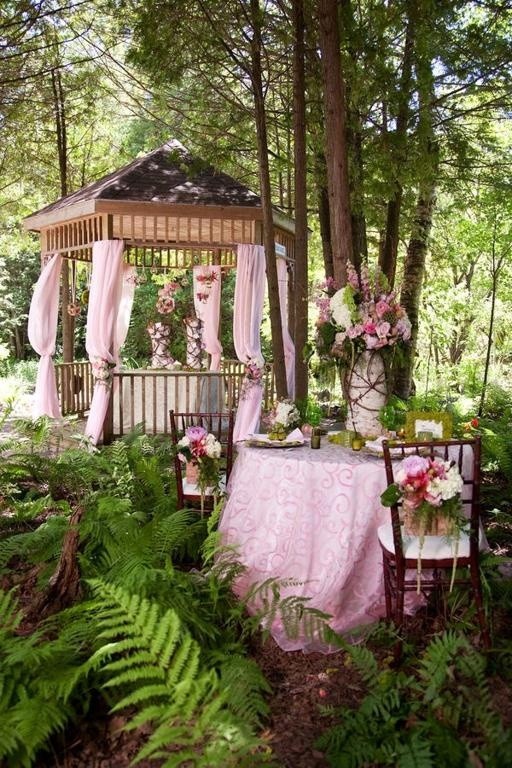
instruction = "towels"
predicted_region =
[251,427,302,444]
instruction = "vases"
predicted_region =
[186,458,201,484]
[402,497,449,536]
[345,351,389,436]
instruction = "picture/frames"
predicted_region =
[405,411,452,443]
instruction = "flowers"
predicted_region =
[303,254,412,406]
[176,426,222,520]
[380,454,476,596]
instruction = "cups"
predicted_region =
[351,439,362,451]
[311,435,320,449]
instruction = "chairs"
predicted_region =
[169,410,233,512]
[376,434,492,666]
